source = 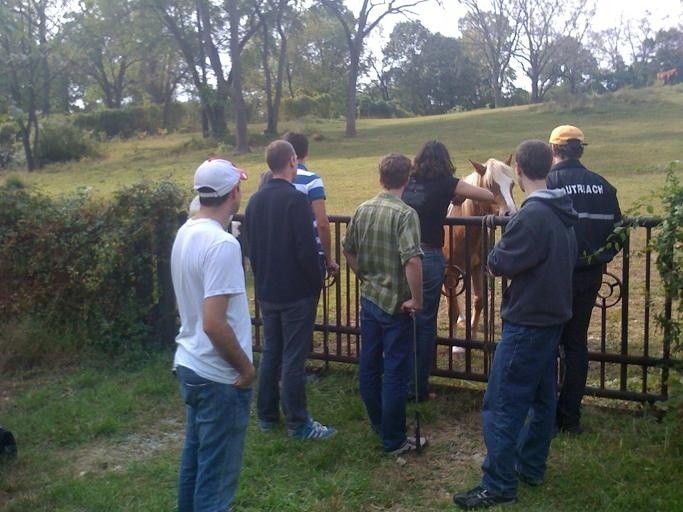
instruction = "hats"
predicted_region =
[193,157,249,198]
[549,125,586,148]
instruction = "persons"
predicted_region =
[172,157,260,511]
[540,126,633,429]
[398,137,492,400]
[240,139,342,440]
[343,153,429,455]
[281,133,341,291]
[451,139,580,509]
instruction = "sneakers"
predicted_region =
[516,466,543,486]
[385,435,427,458]
[454,485,518,509]
[288,419,338,442]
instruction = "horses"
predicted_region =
[442,153,520,361]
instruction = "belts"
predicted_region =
[420,240,437,249]
